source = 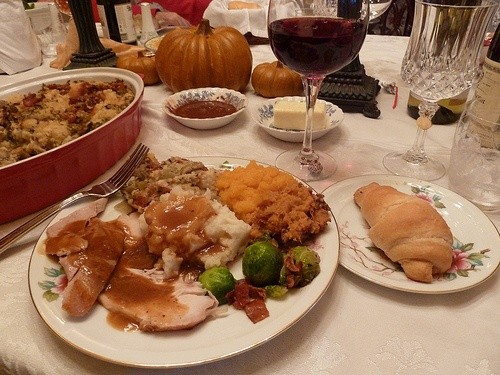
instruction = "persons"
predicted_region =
[133,0,212,26]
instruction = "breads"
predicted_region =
[353,182,455,283]
[228,1,260,9]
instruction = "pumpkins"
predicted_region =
[155,19,252,92]
[250,61,305,98]
[115,50,159,83]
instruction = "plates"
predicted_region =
[163,86,247,130]
[28,155,341,369]
[320,173,500,294]
[253,96,344,143]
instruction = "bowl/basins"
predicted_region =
[0,67,145,225]
[140,25,189,52]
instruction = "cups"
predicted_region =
[446,96,500,215]
[32,11,68,56]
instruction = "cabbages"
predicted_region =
[198,241,321,307]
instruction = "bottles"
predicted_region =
[95,0,138,48]
[406,0,483,126]
[464,22,500,159]
[138,2,161,45]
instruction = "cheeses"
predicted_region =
[272,100,330,131]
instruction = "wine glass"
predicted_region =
[267,0,370,182]
[382,0,497,182]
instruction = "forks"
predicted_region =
[0,144,151,255]
